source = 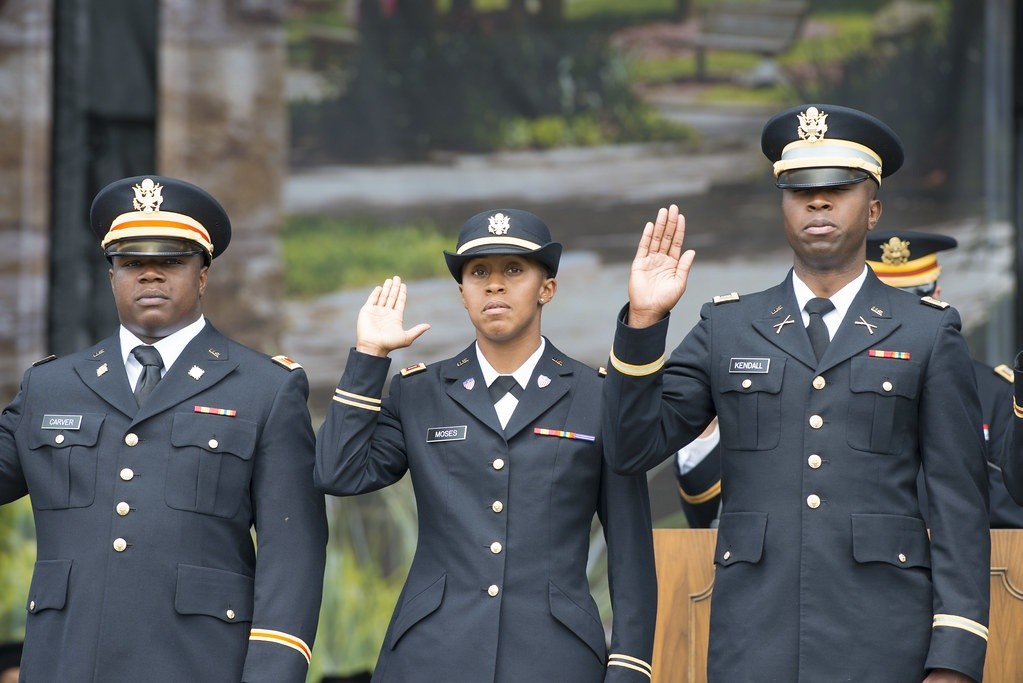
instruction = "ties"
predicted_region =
[130,344,164,412]
[803,297,837,365]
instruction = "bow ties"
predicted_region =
[487,375,523,406]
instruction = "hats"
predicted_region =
[762,104,905,189]
[90,174,232,266]
[443,209,563,288]
[865,229,957,297]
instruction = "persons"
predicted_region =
[313,208,658,682]
[601,104,1022,682]
[1,174,330,683]
